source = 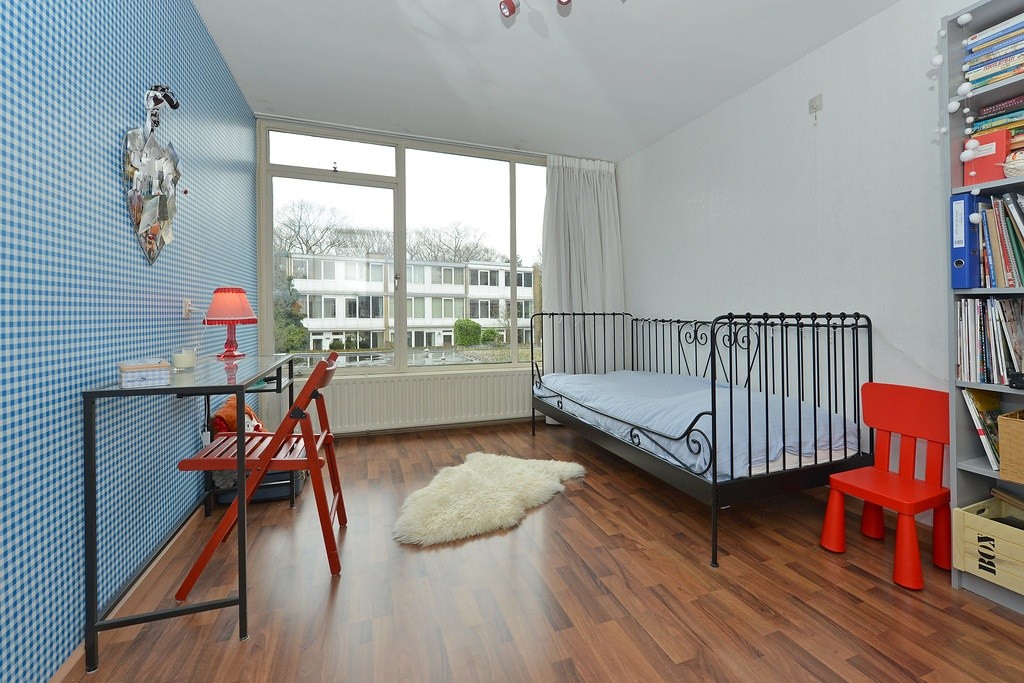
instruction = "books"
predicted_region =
[951,8,1023,470]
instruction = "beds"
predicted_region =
[531,312,873,569]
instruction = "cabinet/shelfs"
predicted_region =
[939,0,1024,614]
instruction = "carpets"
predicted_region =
[397,452,585,546]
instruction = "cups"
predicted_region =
[171,347,196,372]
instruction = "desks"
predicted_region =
[80,354,295,675]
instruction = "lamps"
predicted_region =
[499,0,571,17]
[204,287,258,358]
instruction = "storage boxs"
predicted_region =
[951,498,1024,595]
[118,358,172,389]
[997,408,1024,483]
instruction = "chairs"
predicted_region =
[821,383,948,590]
[173,349,347,601]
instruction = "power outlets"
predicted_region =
[183,299,192,319]
